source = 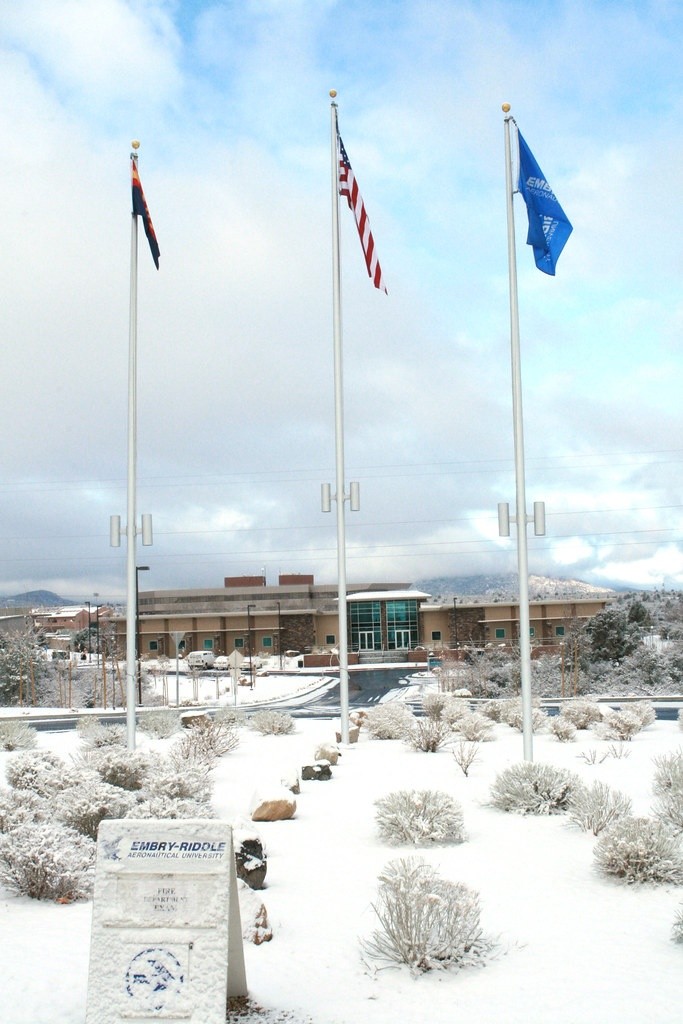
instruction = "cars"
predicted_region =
[214,655,230,671]
[241,658,263,671]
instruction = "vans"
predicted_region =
[188,651,214,670]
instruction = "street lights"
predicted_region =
[276,601,283,671]
[453,597,458,662]
[135,565,151,707]
[96,605,102,652]
[247,604,256,690]
[85,601,92,663]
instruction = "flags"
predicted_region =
[131,161,160,270]
[337,117,389,296]
[509,120,574,276]
